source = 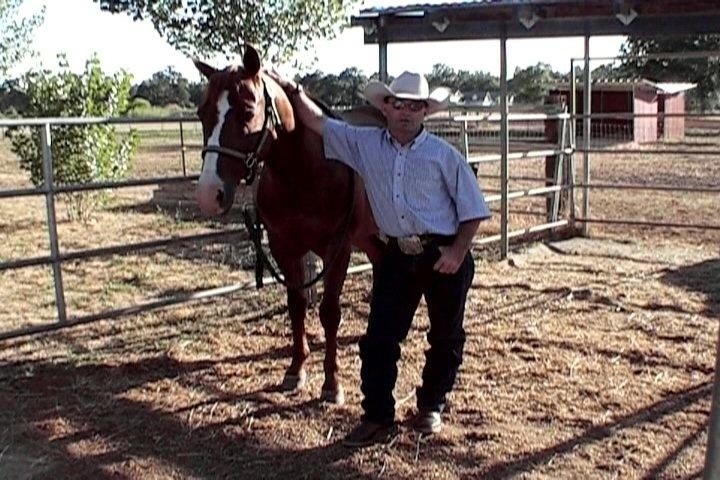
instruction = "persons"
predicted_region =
[267,65,492,448]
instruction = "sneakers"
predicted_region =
[342,422,393,446]
[412,407,441,433]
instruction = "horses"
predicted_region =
[189,42,383,405]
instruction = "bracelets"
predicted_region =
[291,82,303,96]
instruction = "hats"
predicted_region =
[365,71,451,113]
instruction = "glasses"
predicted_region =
[389,101,423,112]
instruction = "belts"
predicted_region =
[377,233,440,256]
